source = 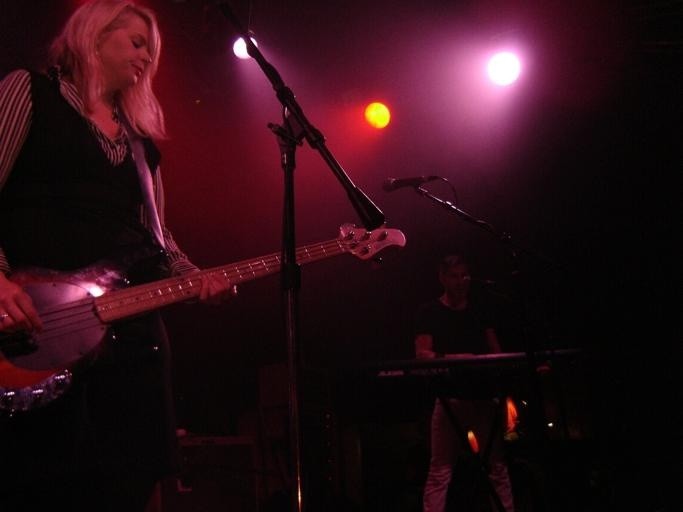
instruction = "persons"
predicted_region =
[410,253,517,511]
[0,2,241,509]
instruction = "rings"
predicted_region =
[0,312,8,321]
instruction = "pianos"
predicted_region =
[376,349,587,386]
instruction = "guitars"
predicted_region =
[0,224,407,412]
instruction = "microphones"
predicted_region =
[381,174,440,193]
